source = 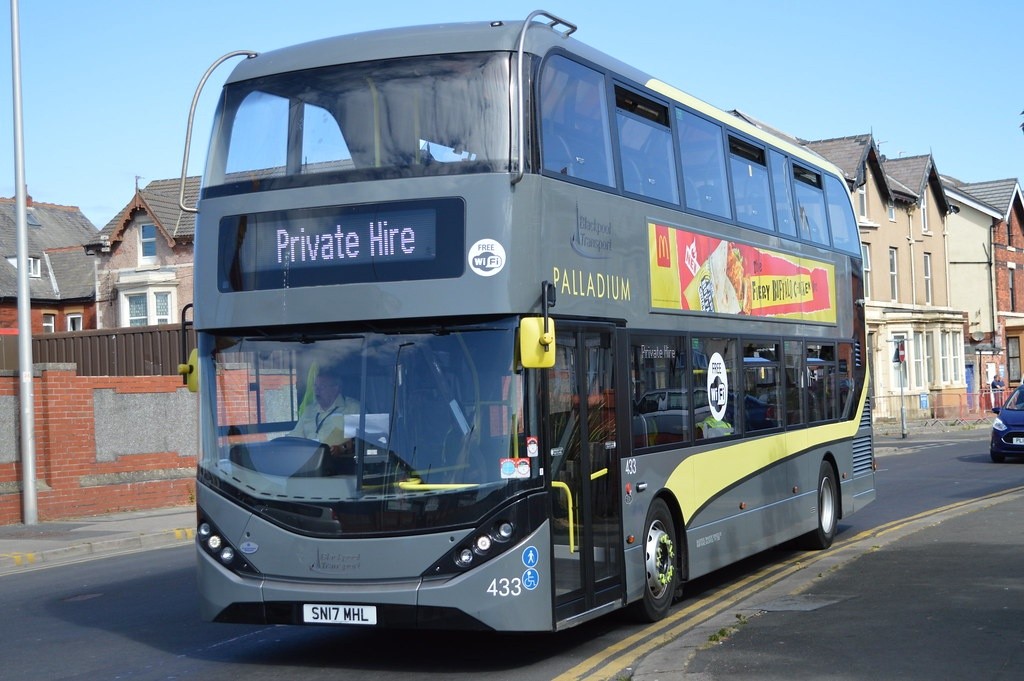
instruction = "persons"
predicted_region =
[991,375,1006,408]
[286,365,367,457]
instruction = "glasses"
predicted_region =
[314,384,336,389]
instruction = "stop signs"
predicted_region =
[899,344,906,362]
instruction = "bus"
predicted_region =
[177,8,876,631]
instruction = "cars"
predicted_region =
[989,386,1023,463]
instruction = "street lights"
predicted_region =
[886,337,912,438]
